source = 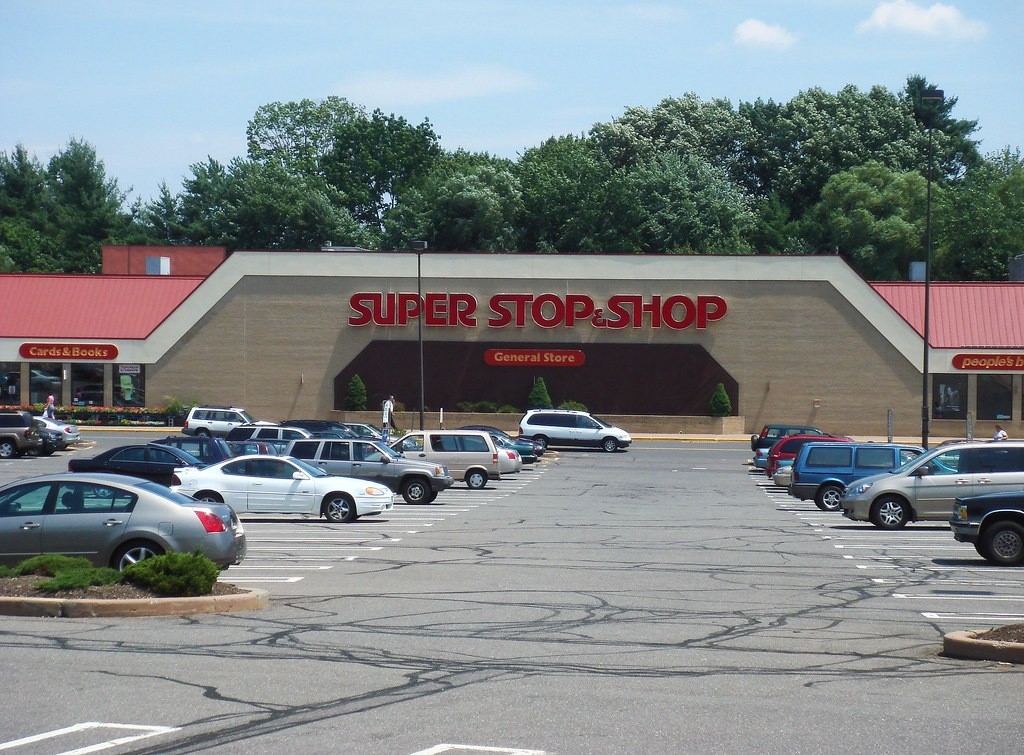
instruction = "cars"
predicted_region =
[33,415,79,451]
[786,442,958,512]
[948,488,1024,566]
[169,454,395,522]
[0,471,247,579]
[1,370,145,407]
[28,424,65,457]
[149,436,232,465]
[753,445,768,471]
[194,441,282,477]
[772,447,913,476]
[838,440,1024,529]
[767,435,856,478]
[438,426,545,465]
[69,444,208,487]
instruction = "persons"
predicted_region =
[994,424,1008,442]
[43,391,55,418]
[73,388,81,401]
[383,395,398,431]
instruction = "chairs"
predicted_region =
[61,492,72,507]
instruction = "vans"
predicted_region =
[519,408,633,452]
[366,429,501,488]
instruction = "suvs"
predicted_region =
[180,405,278,437]
[281,419,376,453]
[0,410,38,458]
[344,421,418,448]
[750,424,833,452]
[225,423,316,442]
[283,438,455,505]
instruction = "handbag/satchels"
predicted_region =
[42,409,48,418]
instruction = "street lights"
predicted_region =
[413,241,428,429]
[920,88,945,452]
